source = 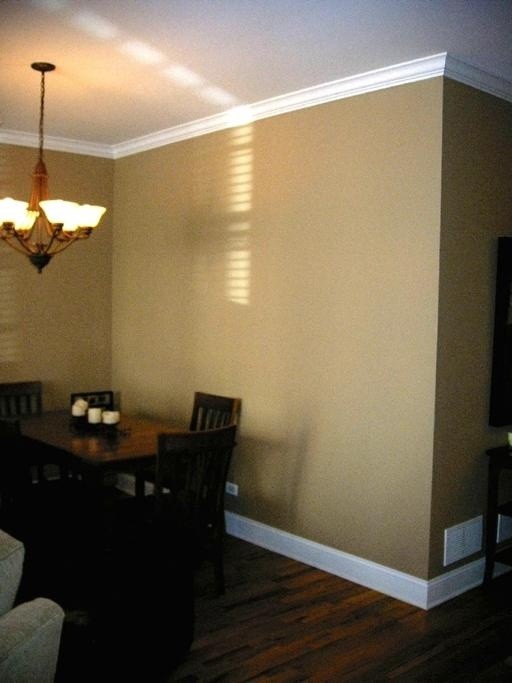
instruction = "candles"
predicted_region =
[113,412,120,423]
[72,405,85,416]
[75,399,88,411]
[87,408,101,424]
[102,411,116,424]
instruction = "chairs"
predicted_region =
[71,390,114,412]
[190,392,241,436]
[116,423,238,589]
[0,381,43,415]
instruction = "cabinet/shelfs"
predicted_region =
[480,445,512,594]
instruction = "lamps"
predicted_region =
[0,63,108,274]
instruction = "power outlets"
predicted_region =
[224,481,239,496]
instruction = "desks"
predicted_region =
[9,408,194,560]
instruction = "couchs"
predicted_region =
[0,531,65,682]
